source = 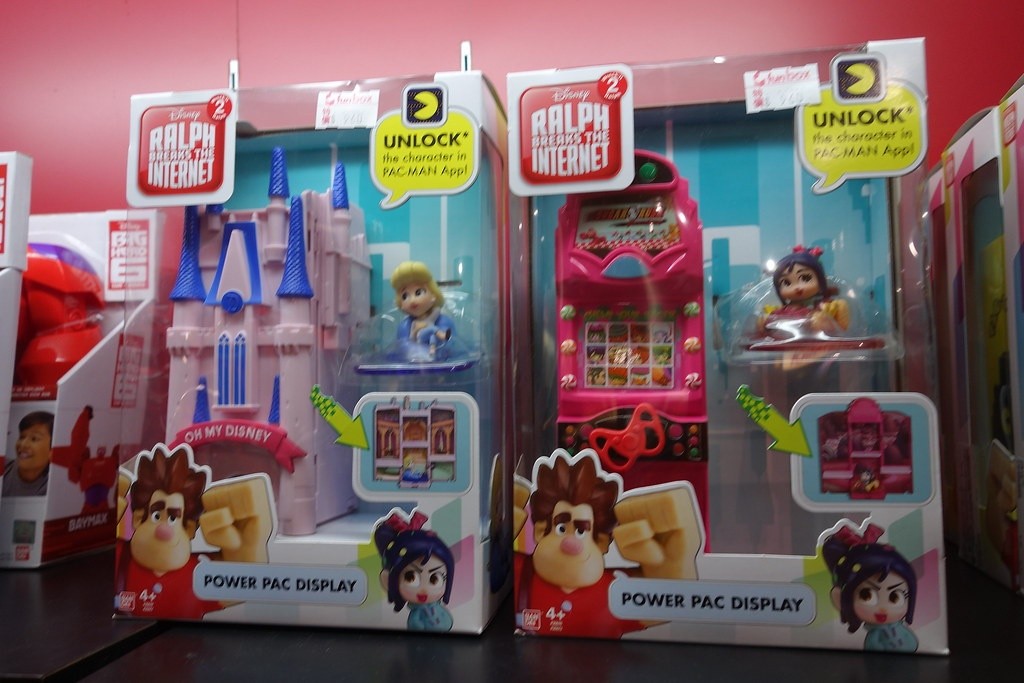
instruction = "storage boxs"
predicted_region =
[505,33,951,658]
[916,75,1024,590]
[111,69,532,633]
[0,152,165,572]
